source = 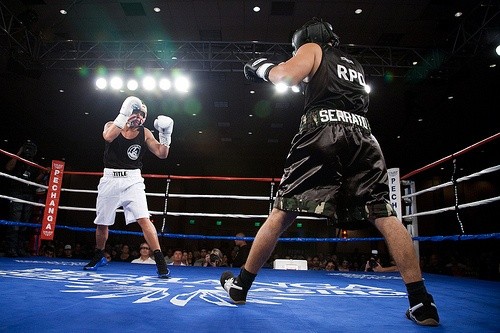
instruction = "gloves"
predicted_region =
[113,96,142,129]
[154,116,174,146]
[244,57,273,82]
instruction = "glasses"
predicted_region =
[140,247,150,250]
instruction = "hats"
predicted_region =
[65,244,72,250]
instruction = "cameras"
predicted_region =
[210,254,218,262]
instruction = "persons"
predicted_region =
[220,16,440,326]
[0,144,462,276]
[84,95,174,276]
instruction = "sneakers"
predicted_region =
[220,271,246,304]
[405,294,439,326]
[84,249,107,271]
[153,249,170,277]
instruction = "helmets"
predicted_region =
[133,101,147,125]
[292,16,340,60]
